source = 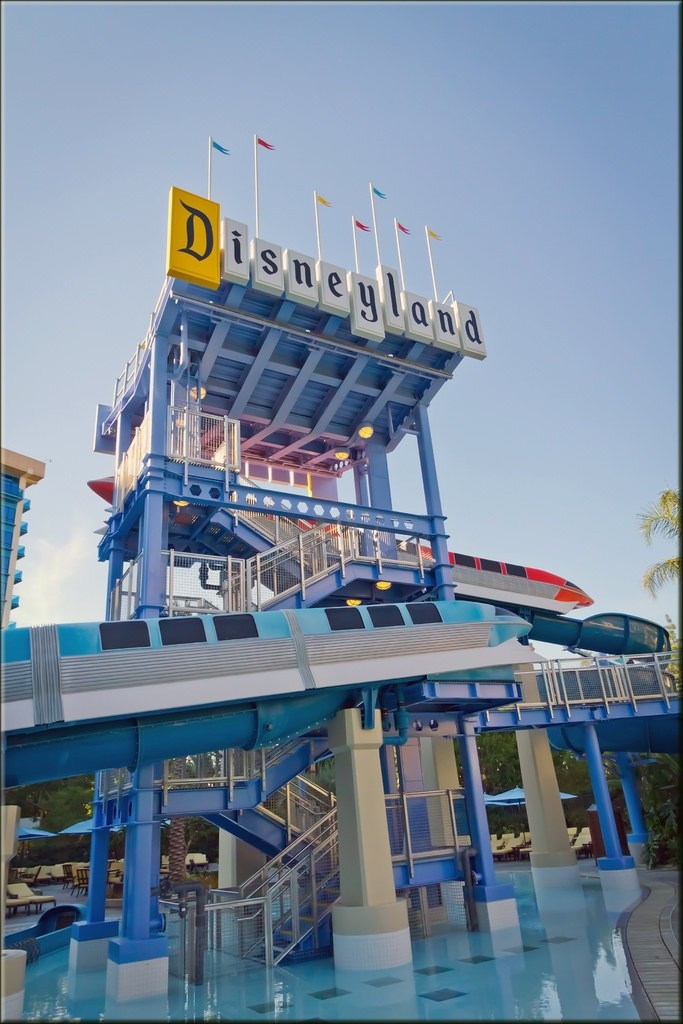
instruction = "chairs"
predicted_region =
[4,853,209,918]
[459,826,593,860]
